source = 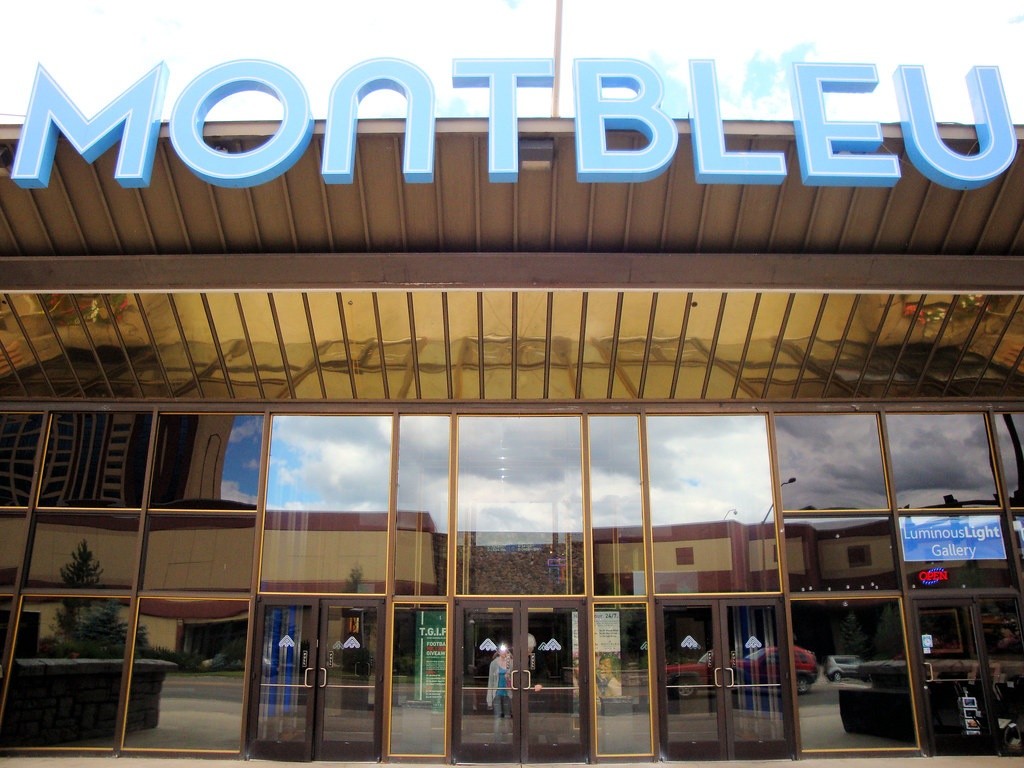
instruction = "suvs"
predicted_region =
[824,655,873,683]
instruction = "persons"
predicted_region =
[595,655,622,699]
[485,646,514,743]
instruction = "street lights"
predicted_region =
[721,508,738,520]
[761,476,800,588]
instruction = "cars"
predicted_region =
[665,645,715,695]
[729,645,818,694]
